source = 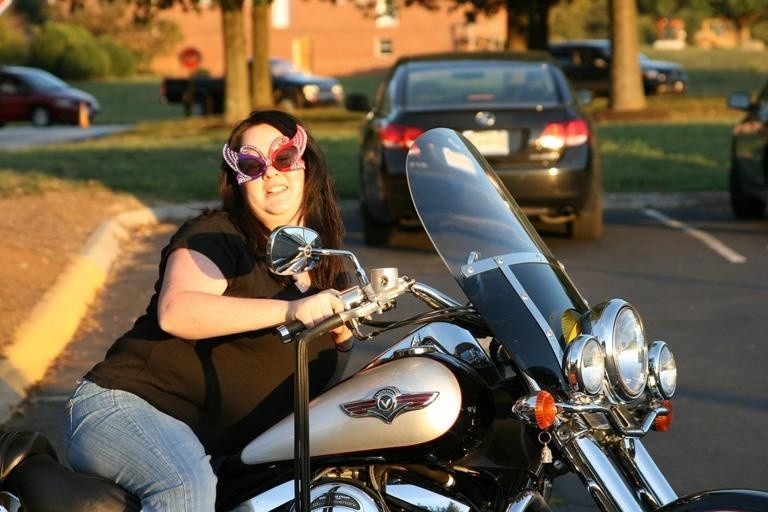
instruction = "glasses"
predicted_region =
[220,123,309,186]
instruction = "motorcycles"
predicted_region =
[2,126,767,510]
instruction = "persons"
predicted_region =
[68,109,356,512]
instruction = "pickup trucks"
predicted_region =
[159,57,346,118]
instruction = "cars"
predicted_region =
[2,65,103,128]
[726,72,767,221]
